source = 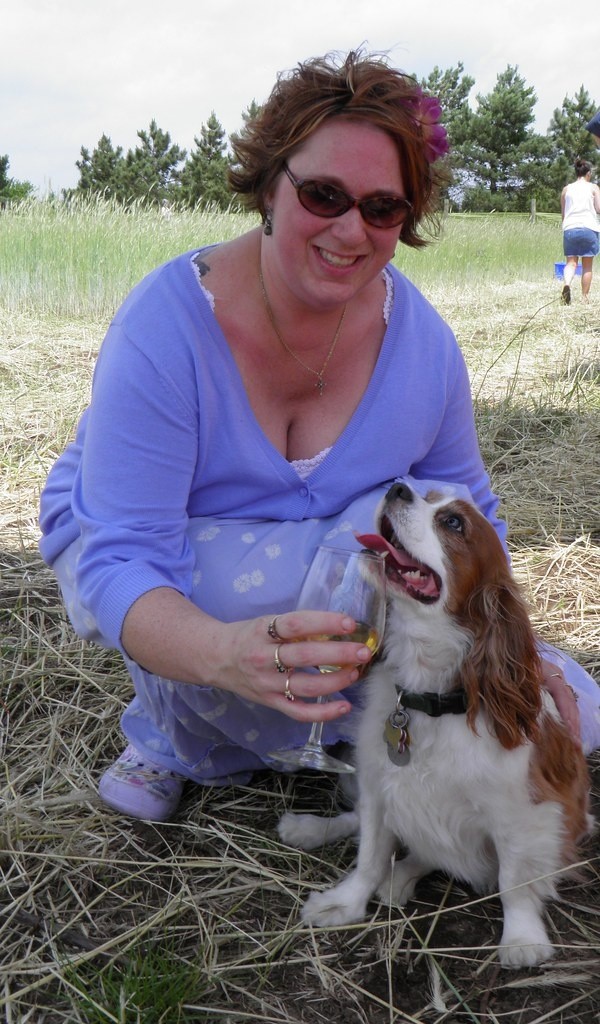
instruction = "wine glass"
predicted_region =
[267,545,386,774]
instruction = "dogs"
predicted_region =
[276,481,599,970]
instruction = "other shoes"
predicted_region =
[99,744,185,820]
[331,739,359,809]
[562,285,572,306]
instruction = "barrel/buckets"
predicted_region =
[555,262,584,276]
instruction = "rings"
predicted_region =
[284,671,297,703]
[551,673,563,681]
[268,615,290,643]
[573,692,578,702]
[273,642,295,675]
[564,683,574,694]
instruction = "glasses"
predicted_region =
[281,161,413,229]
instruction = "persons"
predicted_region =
[161,198,172,220]
[560,159,600,305]
[38,38,599,819]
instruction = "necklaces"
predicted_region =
[257,224,349,395]
